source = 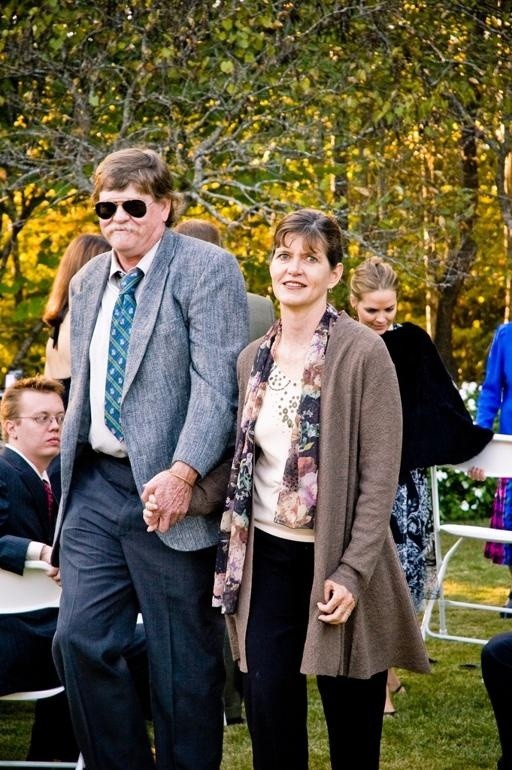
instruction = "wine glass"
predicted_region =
[346,612,350,617]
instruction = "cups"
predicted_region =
[95,200,154,218]
[16,415,64,425]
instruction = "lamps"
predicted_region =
[0,559,88,770]
[420,431,512,646]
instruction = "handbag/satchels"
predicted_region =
[170,470,194,492]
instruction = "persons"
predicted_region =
[469,319,512,618]
[480,633,512,770]
[42,233,111,494]
[51,149,246,770]
[142,209,433,770]
[0,377,149,770]
[173,221,276,723]
[348,257,493,718]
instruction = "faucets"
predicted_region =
[391,683,406,695]
[385,710,398,718]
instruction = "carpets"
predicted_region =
[105,267,145,443]
[42,479,52,516]
